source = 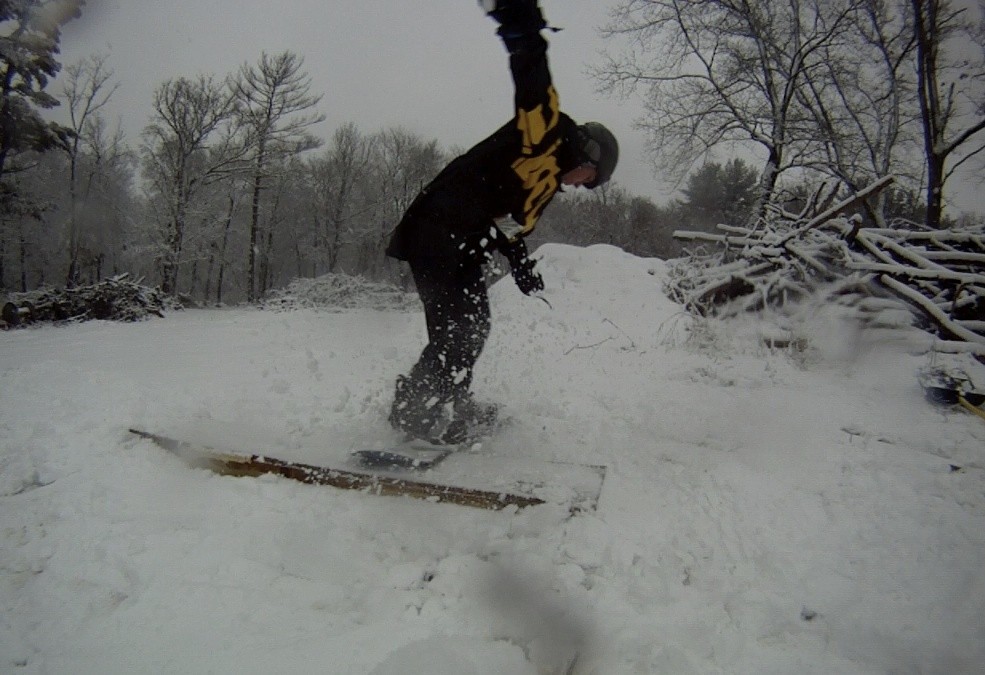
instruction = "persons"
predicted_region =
[385,0,621,452]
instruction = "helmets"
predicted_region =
[576,121,619,190]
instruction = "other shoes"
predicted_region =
[390,384,460,445]
[433,400,498,446]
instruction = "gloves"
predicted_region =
[509,258,544,297]
[488,0,546,35]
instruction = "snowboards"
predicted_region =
[348,404,515,469]
